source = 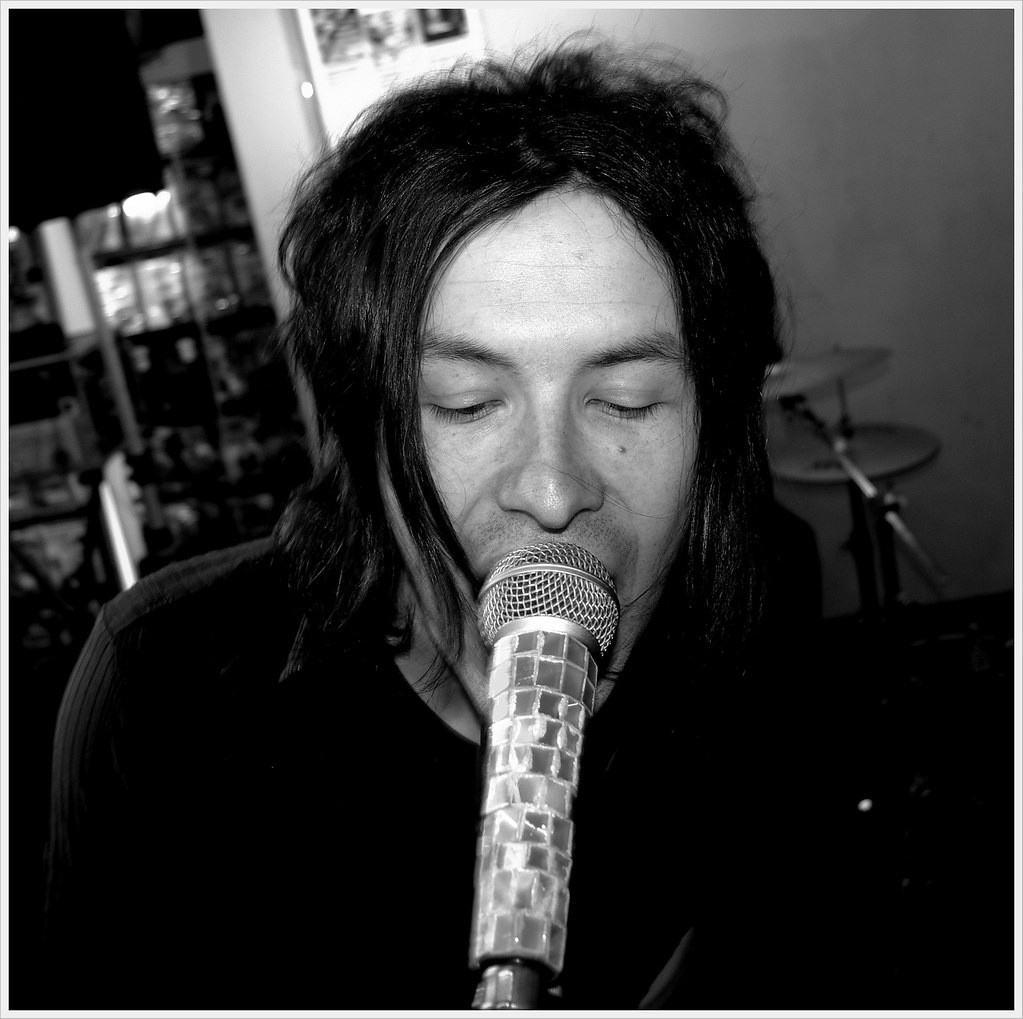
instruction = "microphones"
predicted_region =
[469,542,620,1010]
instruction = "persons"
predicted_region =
[39,58,825,1011]
